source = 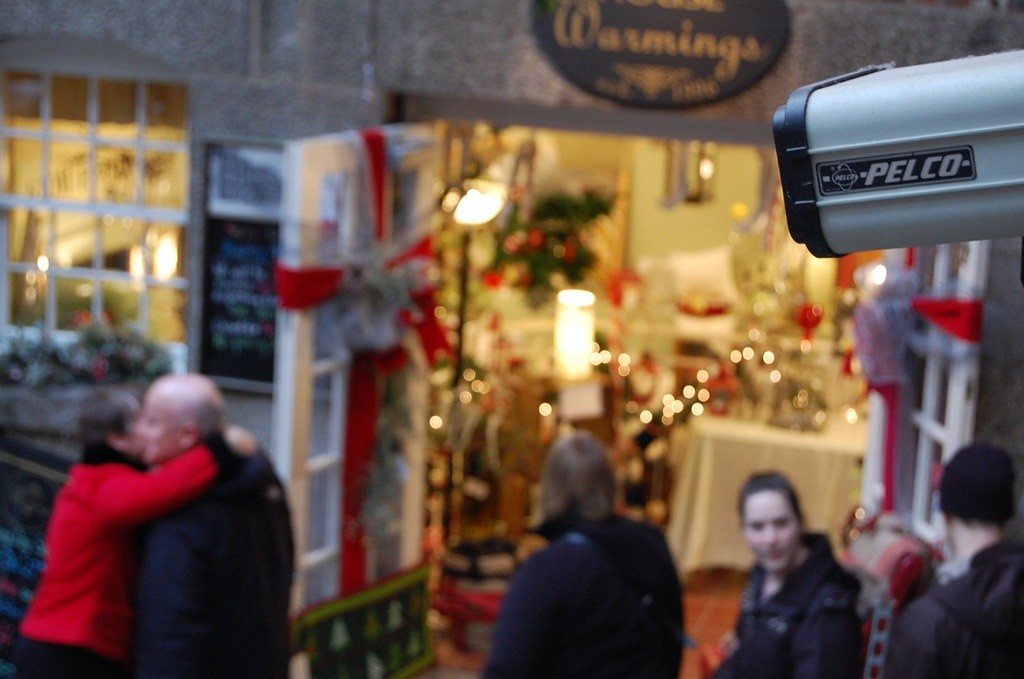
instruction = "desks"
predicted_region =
[663,410,861,589]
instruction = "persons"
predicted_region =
[479,437,683,679]
[879,442,1024,679]
[13,386,261,679]
[710,471,863,679]
[131,371,295,679]
